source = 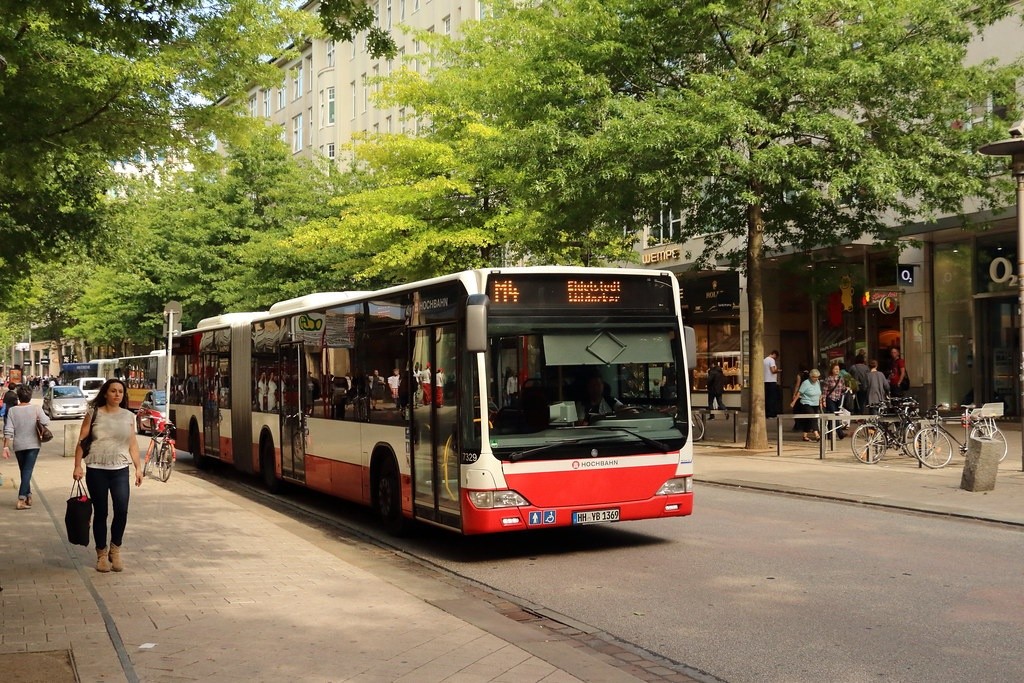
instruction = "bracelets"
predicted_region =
[4,446,8,449]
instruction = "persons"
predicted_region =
[3,384,50,509]
[790,347,910,441]
[171,361,520,414]
[764,349,782,419]
[72,379,143,572]
[0,374,62,426]
[706,360,729,420]
[574,373,637,423]
[660,362,675,407]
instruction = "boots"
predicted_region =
[95,545,110,572]
[109,542,124,572]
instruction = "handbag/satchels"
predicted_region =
[0,403,7,416]
[34,405,53,442]
[840,408,851,428]
[80,407,98,458]
[65,479,92,547]
[900,372,910,391]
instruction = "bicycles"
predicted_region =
[143,422,176,482]
[691,414,705,442]
[292,396,370,462]
[913,402,1007,470]
[850,397,934,465]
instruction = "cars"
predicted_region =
[42,386,90,420]
[72,378,106,402]
[136,390,166,437]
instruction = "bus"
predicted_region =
[161,267,697,536]
[60,350,168,413]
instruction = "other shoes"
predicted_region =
[17,502,32,510]
[840,434,847,440]
[25,497,32,505]
[814,435,820,441]
[803,437,812,441]
[707,417,715,420]
[724,413,729,420]
